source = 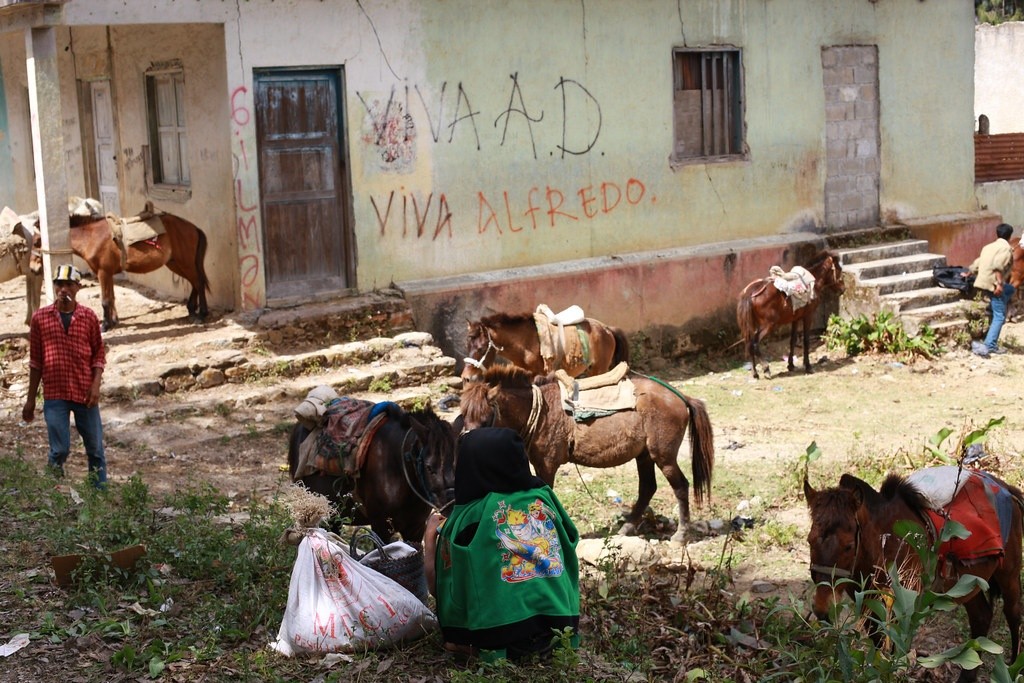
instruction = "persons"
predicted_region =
[22,265,106,494]
[959,223,1016,354]
[424,428,581,662]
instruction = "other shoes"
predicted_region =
[988,348,1006,355]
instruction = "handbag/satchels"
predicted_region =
[270,526,438,658]
[933,265,976,291]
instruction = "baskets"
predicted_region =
[349,527,429,608]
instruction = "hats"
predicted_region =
[52,265,80,284]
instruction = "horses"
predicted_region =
[802,463,1024,683]
[0,196,214,330]
[735,252,846,380]
[285,311,717,548]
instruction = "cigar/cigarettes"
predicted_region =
[66,295,72,302]
